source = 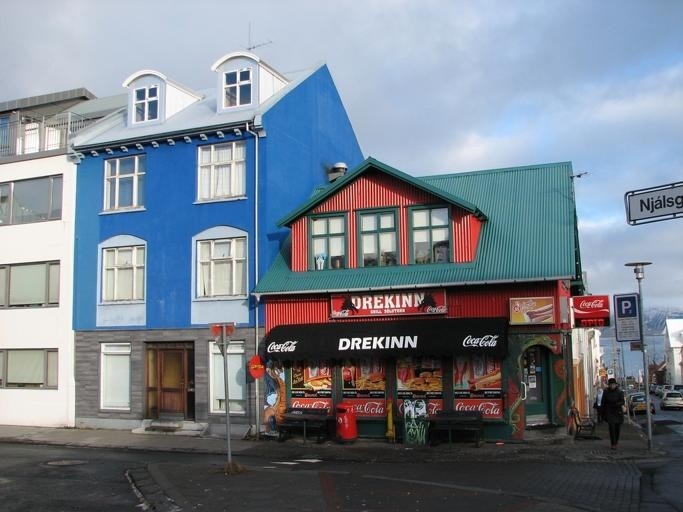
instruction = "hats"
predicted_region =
[608,378,617,385]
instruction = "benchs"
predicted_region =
[277,407,331,444]
[426,410,485,447]
[571,407,596,435]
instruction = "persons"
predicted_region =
[600,378,626,450]
[593,382,626,423]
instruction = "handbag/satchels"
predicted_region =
[593,403,598,409]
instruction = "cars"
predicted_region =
[624,381,683,414]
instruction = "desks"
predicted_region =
[422,416,477,450]
[281,413,329,446]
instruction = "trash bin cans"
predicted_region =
[335,403,358,444]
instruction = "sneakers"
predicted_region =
[611,444,618,449]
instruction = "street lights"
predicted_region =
[624,261,654,452]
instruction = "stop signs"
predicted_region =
[248,354,266,381]
